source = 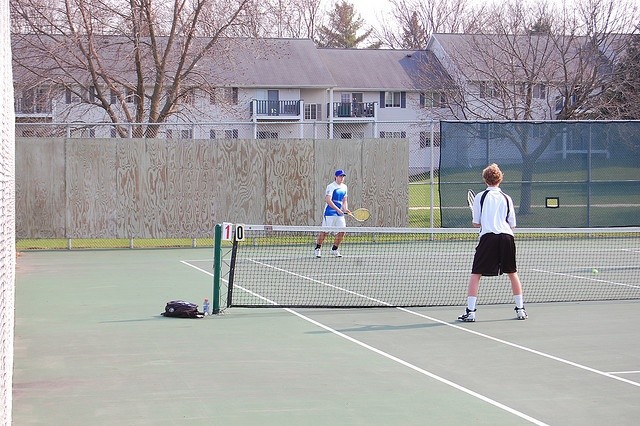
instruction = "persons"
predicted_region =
[457,164,527,322]
[315,171,351,258]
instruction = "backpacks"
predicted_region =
[161,300,204,318]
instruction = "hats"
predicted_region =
[335,169,346,176]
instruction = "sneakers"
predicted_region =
[315,248,321,258]
[330,250,342,257]
[458,307,476,322]
[514,305,528,320]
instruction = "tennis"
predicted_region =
[592,268,598,275]
